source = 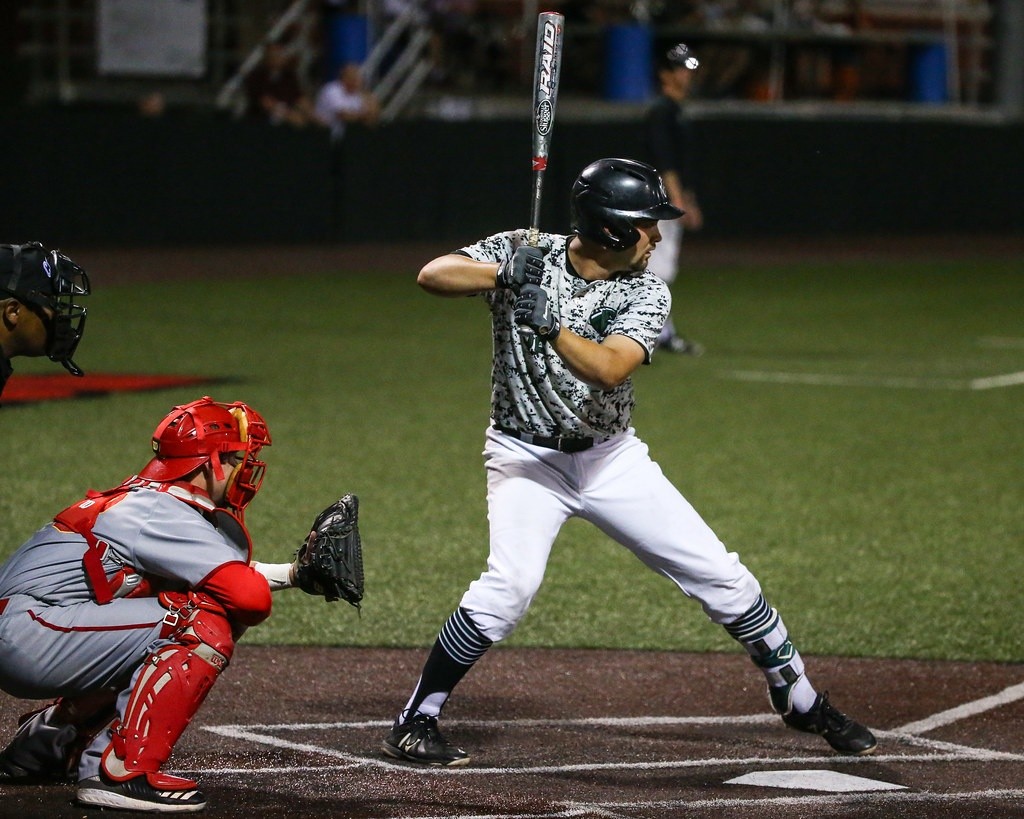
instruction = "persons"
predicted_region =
[630,43,702,357]
[0,0,1024,124]
[0,397,335,812]
[380,157,878,767]
[0,240,92,395]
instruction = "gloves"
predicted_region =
[496,246,551,289]
[513,277,562,341]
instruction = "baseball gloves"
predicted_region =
[293,491,367,622]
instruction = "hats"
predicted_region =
[0,242,57,303]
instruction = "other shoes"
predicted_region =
[664,336,698,356]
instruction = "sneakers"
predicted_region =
[782,699,877,757]
[1,751,84,787]
[382,711,473,766]
[78,772,206,812]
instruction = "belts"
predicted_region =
[498,422,595,453]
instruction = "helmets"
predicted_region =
[569,158,688,250]
[658,42,695,70]
[140,403,240,483]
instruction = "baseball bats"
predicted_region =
[516,10,564,337]
